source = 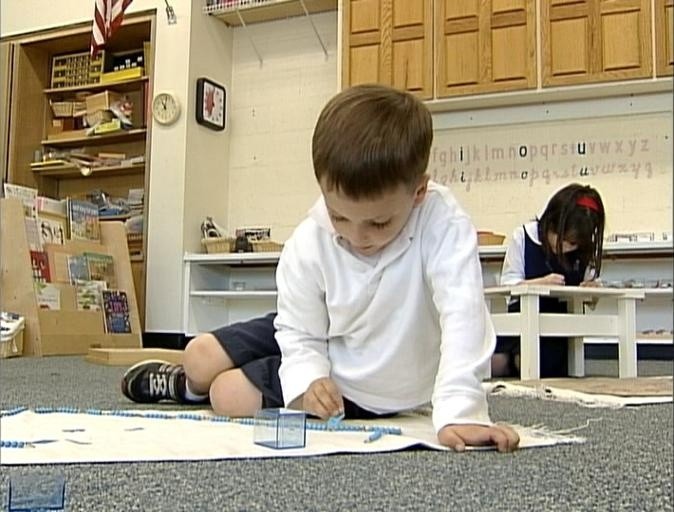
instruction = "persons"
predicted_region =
[120,80,521,456]
[491,181,606,378]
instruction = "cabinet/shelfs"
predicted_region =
[339,0,541,113]
[1,196,145,358]
[183,241,674,347]
[6,13,156,334]
[538,0,674,101]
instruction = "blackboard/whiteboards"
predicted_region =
[424,109,674,246]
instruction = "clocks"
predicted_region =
[151,91,181,125]
[195,77,226,131]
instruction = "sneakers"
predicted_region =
[121,359,210,405]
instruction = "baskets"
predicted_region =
[201,228,235,255]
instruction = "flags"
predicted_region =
[90,0,133,61]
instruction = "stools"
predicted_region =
[483,283,648,381]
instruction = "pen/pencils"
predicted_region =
[546,261,554,273]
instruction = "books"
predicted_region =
[2,182,145,335]
[29,148,146,172]
